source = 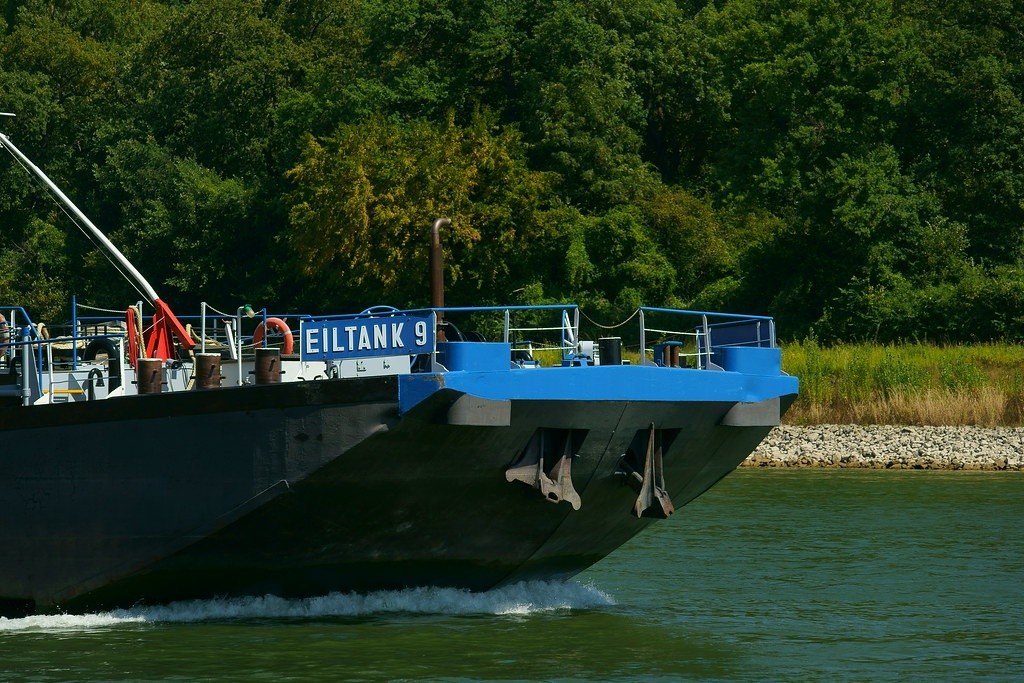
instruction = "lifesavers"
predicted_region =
[0,313,10,358]
[254,317,294,354]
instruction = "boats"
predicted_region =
[2,110,799,620]
